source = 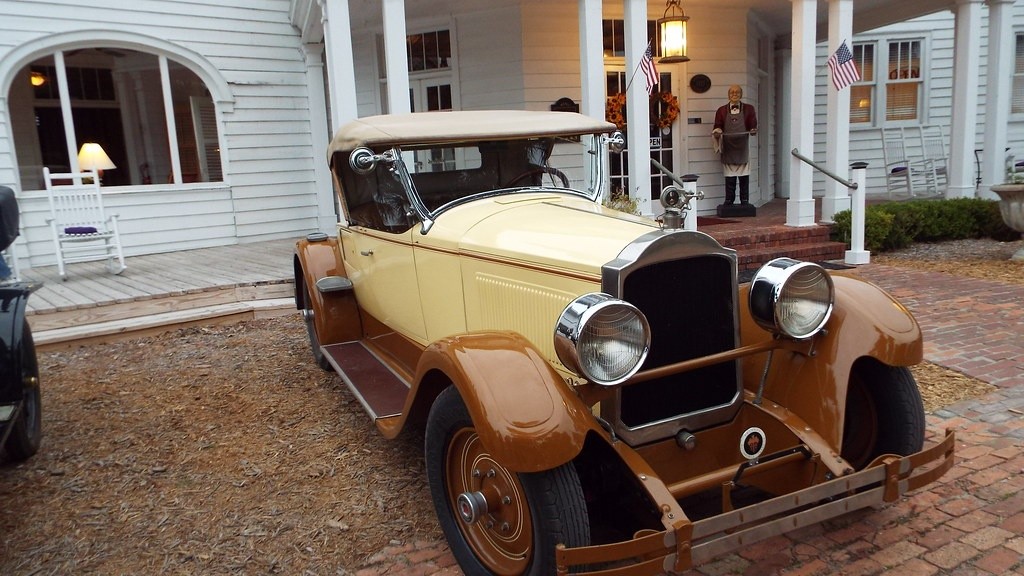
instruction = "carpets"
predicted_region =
[682,216,742,227]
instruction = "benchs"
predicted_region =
[372,170,500,220]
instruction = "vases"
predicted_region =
[990,183,1024,259]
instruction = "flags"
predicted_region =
[640,44,660,95]
[827,42,861,91]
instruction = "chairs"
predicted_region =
[42,166,127,280]
[168,172,200,183]
[879,125,941,198]
[920,125,952,195]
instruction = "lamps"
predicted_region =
[30,68,45,86]
[78,142,117,185]
[657,0,691,65]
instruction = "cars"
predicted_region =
[0,183,43,472]
[292,108,959,576]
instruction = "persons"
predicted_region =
[711,84,758,207]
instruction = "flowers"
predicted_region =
[1002,164,1024,185]
[608,92,680,130]
